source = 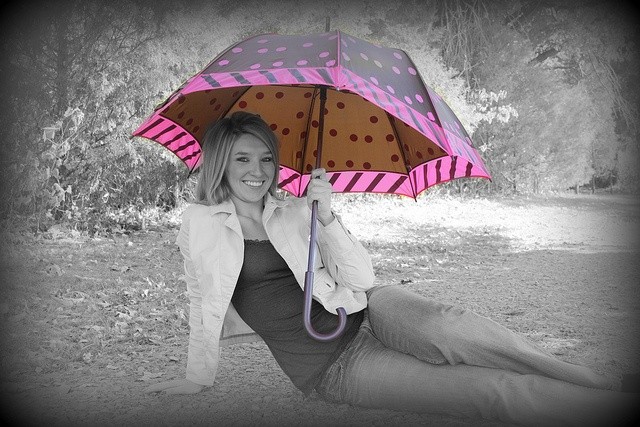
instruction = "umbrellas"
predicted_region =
[129,16,492,342]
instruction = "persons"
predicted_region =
[143,111,640,427]
